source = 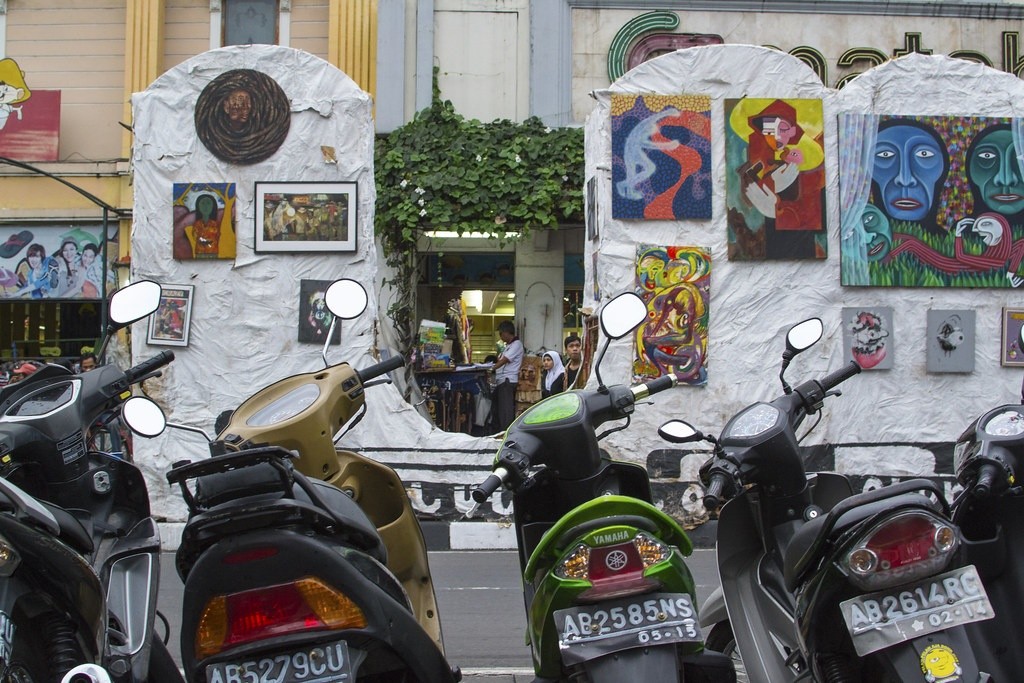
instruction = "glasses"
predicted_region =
[500,331,504,336]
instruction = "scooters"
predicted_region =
[164,277,462,683]
[657,316,995,683]
[0,278,185,683]
[471,292,734,683]
[949,379,1024,681]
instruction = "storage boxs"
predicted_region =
[417,319,450,354]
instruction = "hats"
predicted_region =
[14,364,37,374]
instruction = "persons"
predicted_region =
[541,350,567,399]
[5,239,104,300]
[491,321,525,431]
[565,336,586,391]
[7,364,37,385]
[81,353,124,459]
[484,355,498,395]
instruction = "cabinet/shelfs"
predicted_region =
[467,313,515,364]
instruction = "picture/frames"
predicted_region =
[254,181,359,252]
[1001,306,1024,367]
[145,283,195,348]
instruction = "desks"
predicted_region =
[418,371,488,435]
